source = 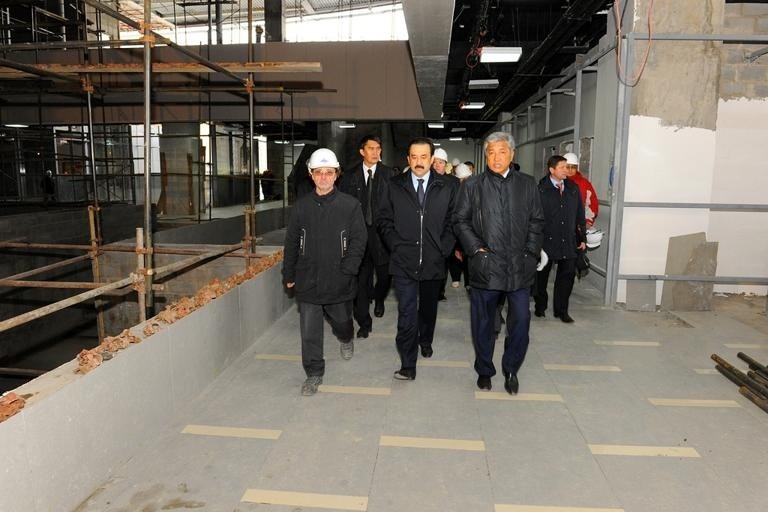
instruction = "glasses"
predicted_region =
[566,165,577,169]
[313,169,336,177]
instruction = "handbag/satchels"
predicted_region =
[575,244,590,280]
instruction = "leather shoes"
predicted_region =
[535,310,545,318]
[500,314,506,324]
[394,370,416,379]
[554,312,575,323]
[357,324,372,338]
[477,375,491,391]
[495,331,498,338]
[374,302,384,317]
[421,345,434,357]
[504,372,518,395]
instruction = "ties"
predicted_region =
[366,168,373,226]
[417,178,425,207]
[556,182,562,197]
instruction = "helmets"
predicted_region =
[585,226,603,248]
[456,163,472,178]
[537,250,549,271]
[434,148,448,163]
[308,148,339,174]
[452,158,459,165]
[563,153,578,165]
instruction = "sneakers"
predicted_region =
[452,281,459,288]
[341,340,354,360]
[302,375,322,395]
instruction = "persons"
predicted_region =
[456,163,475,294]
[338,135,397,339]
[564,152,598,227]
[451,130,544,395]
[433,148,463,289]
[451,158,460,175]
[378,138,461,384]
[465,161,478,174]
[532,156,587,323]
[283,150,369,398]
